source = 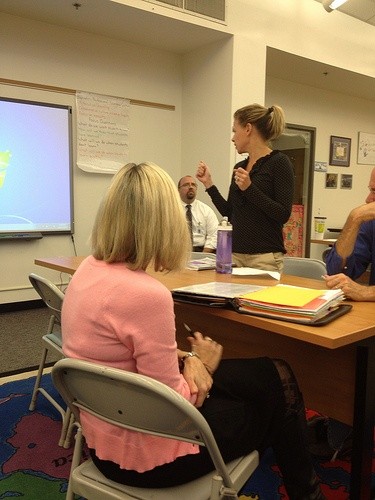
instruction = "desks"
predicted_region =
[35,255,375,500]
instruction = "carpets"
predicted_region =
[0,373,375,500]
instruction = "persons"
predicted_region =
[178,175,221,254]
[61,161,326,500]
[324,165,375,302]
[196,103,295,273]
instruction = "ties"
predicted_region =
[186,205,193,245]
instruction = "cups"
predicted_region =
[314,216,326,241]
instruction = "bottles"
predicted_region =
[215,216,233,274]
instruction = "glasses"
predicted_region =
[179,183,197,188]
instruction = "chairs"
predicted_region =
[29,273,260,500]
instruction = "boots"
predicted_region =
[270,392,327,500]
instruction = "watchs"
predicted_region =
[181,351,200,366]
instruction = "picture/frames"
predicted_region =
[325,173,338,189]
[340,173,352,189]
[330,135,351,167]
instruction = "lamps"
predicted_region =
[323,0,349,13]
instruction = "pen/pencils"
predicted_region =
[184,322,196,338]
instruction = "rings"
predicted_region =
[206,393,210,397]
[236,176,239,181]
[206,337,212,341]
[336,286,338,289]
